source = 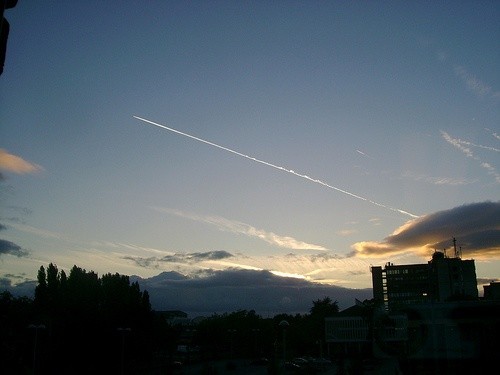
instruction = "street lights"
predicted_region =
[279,320,290,361]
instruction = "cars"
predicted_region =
[280,358,309,369]
